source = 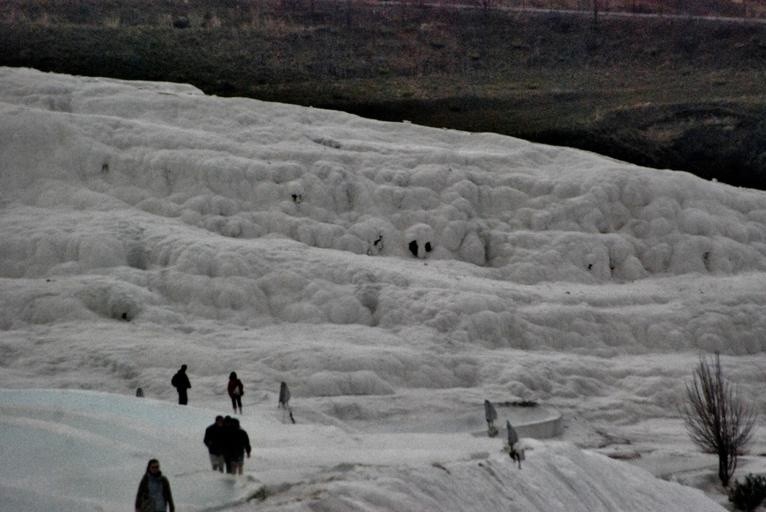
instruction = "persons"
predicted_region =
[227,372,244,409]
[136,459,174,512]
[172,365,191,404]
[223,416,236,474]
[230,419,251,475]
[205,416,224,474]
